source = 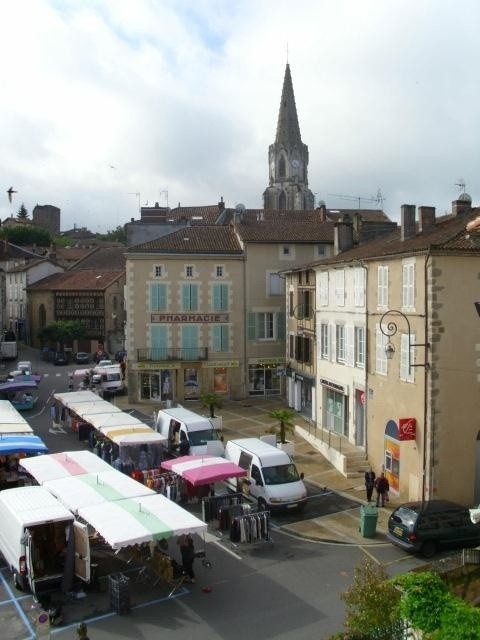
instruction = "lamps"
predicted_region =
[294,304,317,340]
[381,310,430,375]
[198,390,223,431]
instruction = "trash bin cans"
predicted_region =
[358,505,378,538]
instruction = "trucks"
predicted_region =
[100,364,127,395]
[154,403,224,462]
[219,437,310,511]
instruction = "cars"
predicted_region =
[183,380,199,389]
[39,345,126,381]
[7,360,32,386]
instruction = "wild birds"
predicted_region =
[6,187,18,203]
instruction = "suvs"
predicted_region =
[386,499,477,559]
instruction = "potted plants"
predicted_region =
[77,622,90,640]
[266,408,295,457]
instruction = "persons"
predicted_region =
[83,372,91,387]
[374,472,390,506]
[24,393,33,402]
[364,469,376,501]
[68,376,74,389]
[197,485,205,504]
[176,533,200,584]
[210,483,215,496]
